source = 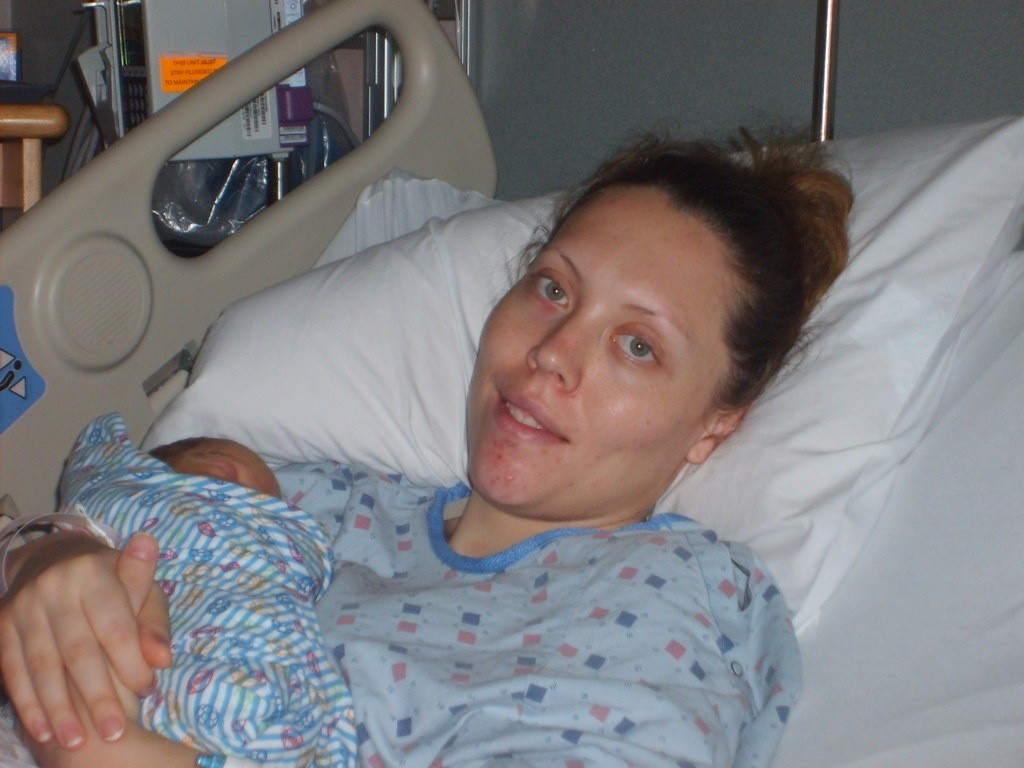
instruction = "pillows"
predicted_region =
[137,117,1024,639]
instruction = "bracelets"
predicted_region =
[0,513,110,594]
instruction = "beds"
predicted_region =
[0,0,1024,768]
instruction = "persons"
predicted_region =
[58,409,357,768]
[0,128,854,768]
